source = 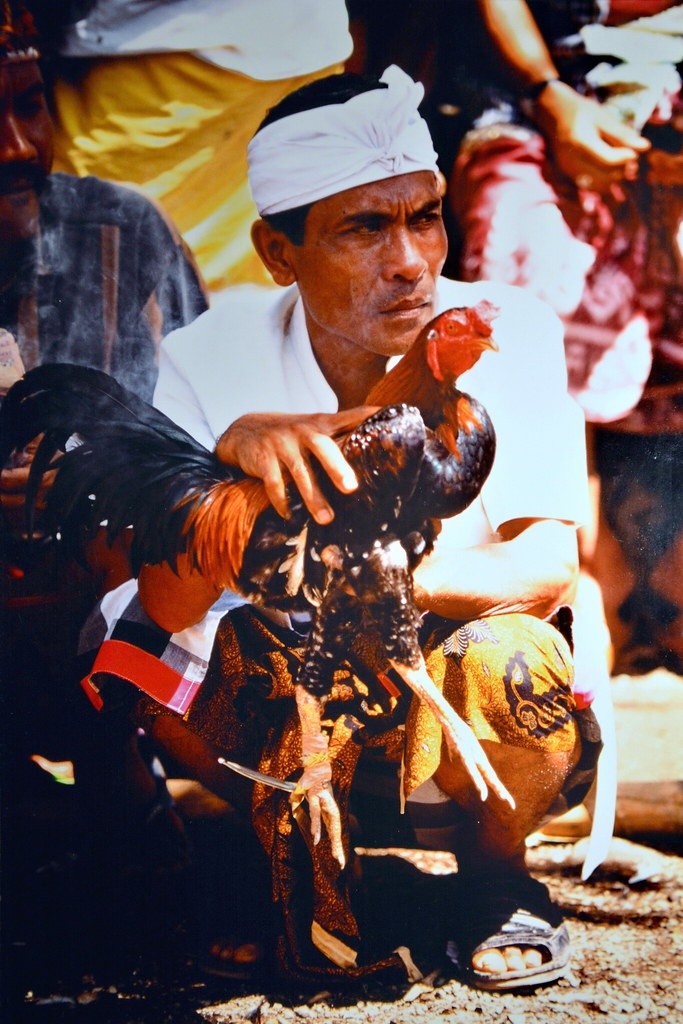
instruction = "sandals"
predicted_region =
[469,908,573,991]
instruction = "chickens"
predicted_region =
[0,298,518,869]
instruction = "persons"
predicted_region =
[0,0,683,989]
